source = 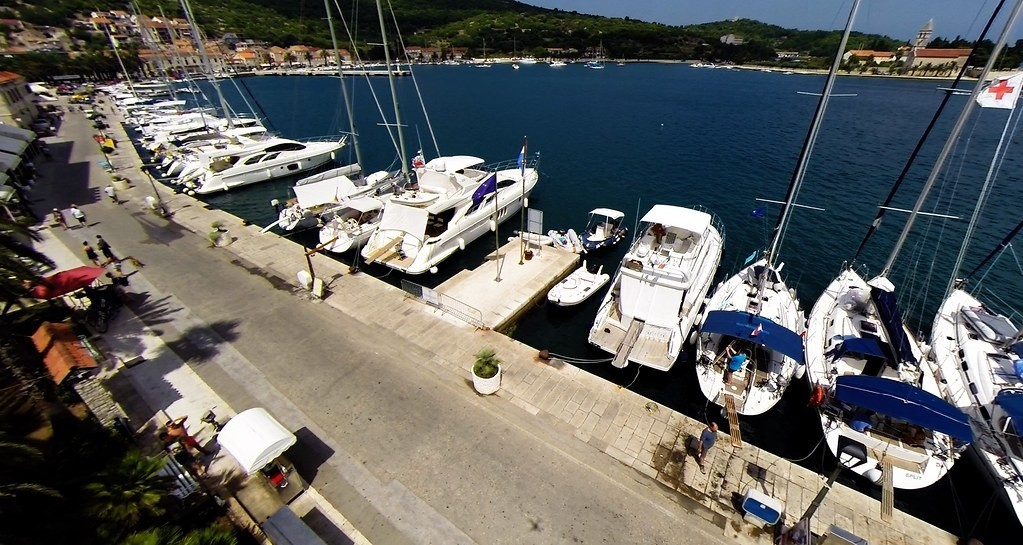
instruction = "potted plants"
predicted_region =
[208,220,233,248]
[109,175,132,192]
[471,348,501,395]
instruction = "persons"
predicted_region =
[651,224,665,243]
[83,234,115,266]
[70,204,88,227]
[53,208,68,231]
[697,421,718,474]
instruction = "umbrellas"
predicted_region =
[33,267,104,300]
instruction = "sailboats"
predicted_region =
[805,0,1023,522]
[443,36,606,70]
[92,1,350,194]
[696,0,861,416]
[271,1,441,253]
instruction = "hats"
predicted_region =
[52,208,58,211]
[70,204,77,208]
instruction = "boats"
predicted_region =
[582,208,627,248]
[690,62,794,75]
[617,63,625,66]
[362,122,543,275]
[508,231,553,245]
[547,261,610,305]
[588,198,725,371]
[549,230,583,254]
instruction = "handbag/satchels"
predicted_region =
[54,214,58,222]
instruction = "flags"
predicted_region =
[976,70,1023,109]
[749,206,767,219]
[518,144,525,176]
[471,173,497,205]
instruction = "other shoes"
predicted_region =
[699,464,706,474]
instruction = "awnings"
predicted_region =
[217,407,297,475]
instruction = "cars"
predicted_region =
[55,83,96,104]
